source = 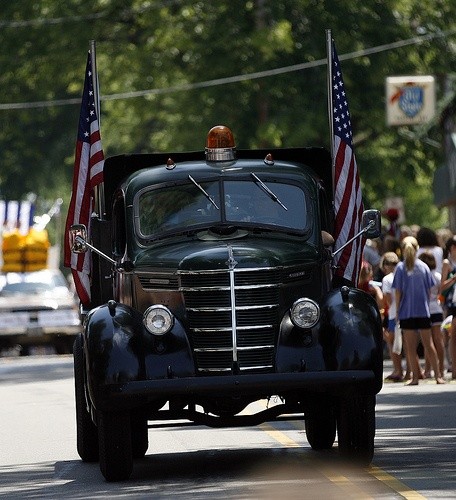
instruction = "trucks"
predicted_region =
[65,28,385,482]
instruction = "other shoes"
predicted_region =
[386,376,402,383]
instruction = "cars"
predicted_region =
[0,266,83,355]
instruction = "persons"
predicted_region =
[357,262,384,310]
[359,222,456,387]
[241,185,335,248]
[391,235,447,387]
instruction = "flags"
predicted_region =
[63,38,108,312]
[323,29,365,289]
